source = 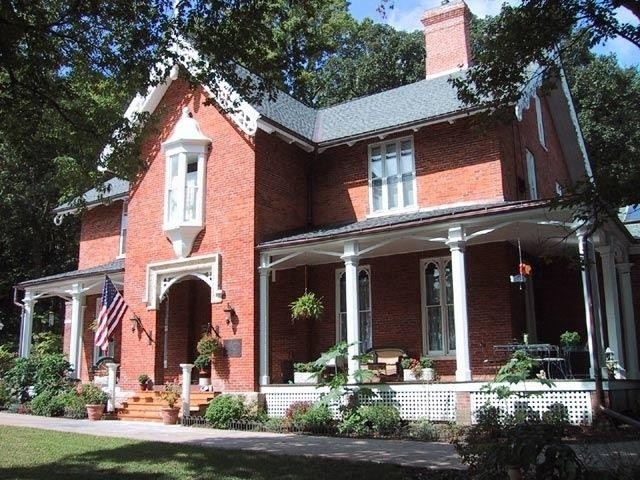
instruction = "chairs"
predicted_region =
[477,336,577,381]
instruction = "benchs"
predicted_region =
[361,347,406,381]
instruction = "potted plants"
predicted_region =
[293,363,323,383]
[289,291,325,325]
[401,355,437,382]
[85,390,111,420]
[156,381,186,424]
[139,375,149,390]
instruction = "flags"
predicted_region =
[95,274,129,357]
